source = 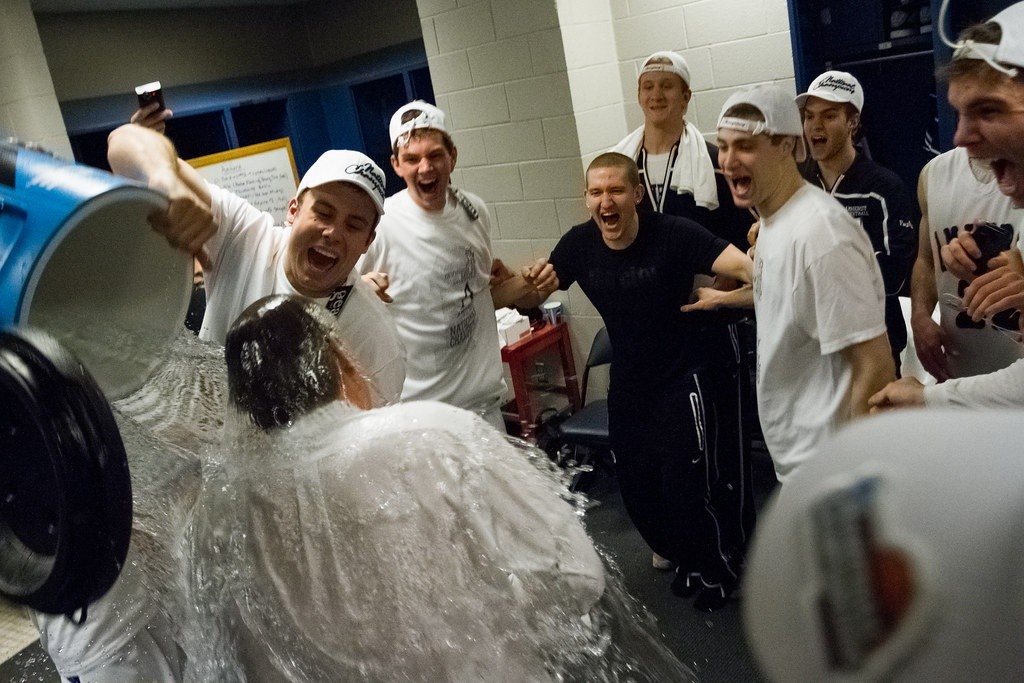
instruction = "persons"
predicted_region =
[792,69,920,381]
[606,50,765,578]
[357,99,560,438]
[25,102,606,683]
[487,150,752,613]
[866,0,1024,421]
[680,82,903,491]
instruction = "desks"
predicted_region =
[499,321,581,453]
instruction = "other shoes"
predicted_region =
[652,551,672,569]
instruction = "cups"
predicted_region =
[544,301,562,325]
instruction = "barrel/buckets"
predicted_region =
[0,139,194,397]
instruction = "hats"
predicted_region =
[936,0,1024,77]
[295,150,386,226]
[793,71,864,116]
[638,51,691,90]
[389,100,451,154]
[739,404,1021,681]
[716,84,806,161]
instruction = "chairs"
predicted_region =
[561,326,617,494]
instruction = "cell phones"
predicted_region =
[135,80,165,117]
